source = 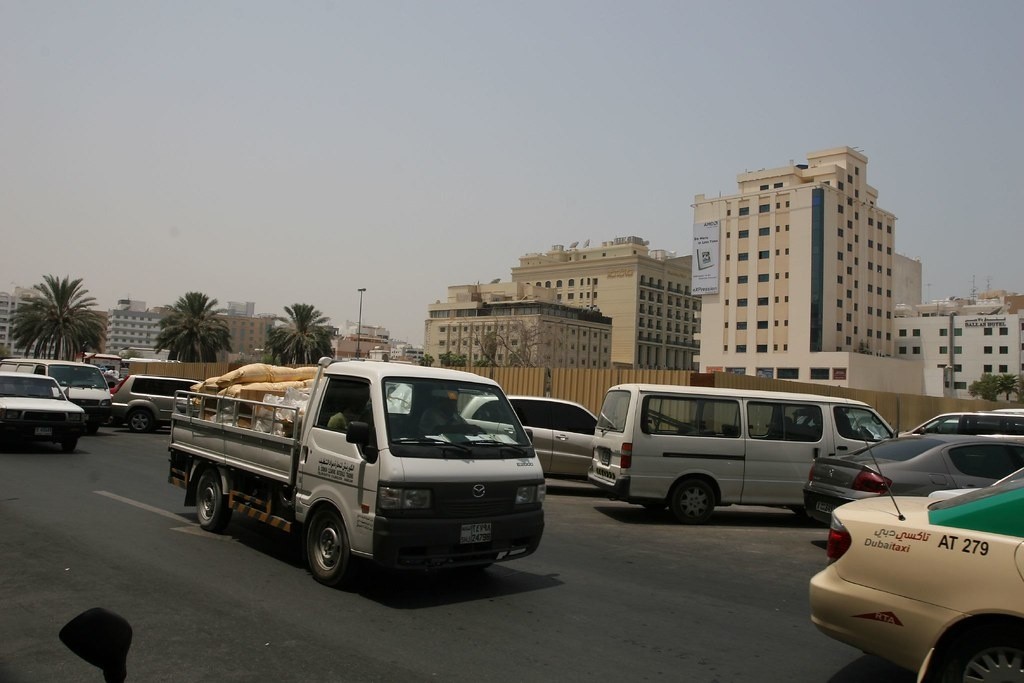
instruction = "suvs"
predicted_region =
[110,374,206,433]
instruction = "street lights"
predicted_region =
[357,288,366,360]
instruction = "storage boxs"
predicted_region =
[239,388,285,428]
[205,384,222,420]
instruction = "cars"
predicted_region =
[97,370,125,427]
[459,394,596,479]
[802,433,1024,525]
[0,371,87,453]
[896,410,1024,435]
[808,466,1024,683]
[794,414,890,437]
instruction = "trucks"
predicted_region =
[167,356,546,589]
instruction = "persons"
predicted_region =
[324,382,374,435]
[15,379,27,393]
[419,387,486,435]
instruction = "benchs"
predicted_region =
[773,421,816,441]
[721,423,739,436]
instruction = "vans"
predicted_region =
[587,383,896,523]
[0,358,113,436]
